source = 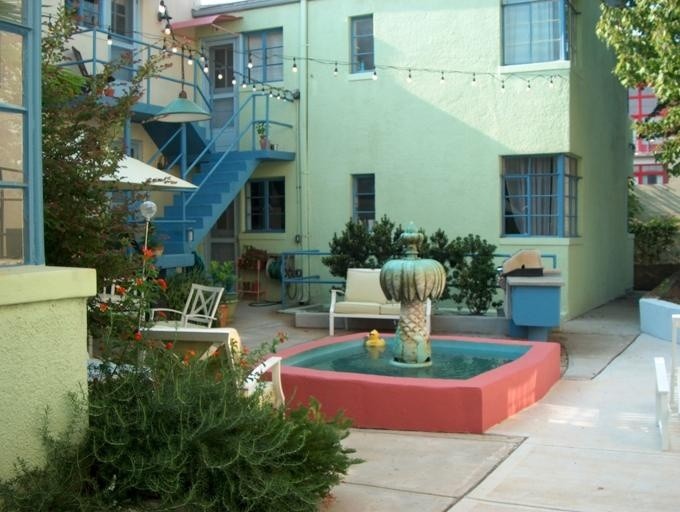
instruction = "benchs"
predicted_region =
[326,266,432,336]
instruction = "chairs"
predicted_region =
[136,329,287,411]
[148,282,225,330]
[652,314,680,453]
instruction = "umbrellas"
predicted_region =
[68,137,202,192]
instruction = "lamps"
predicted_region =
[152,46,213,125]
